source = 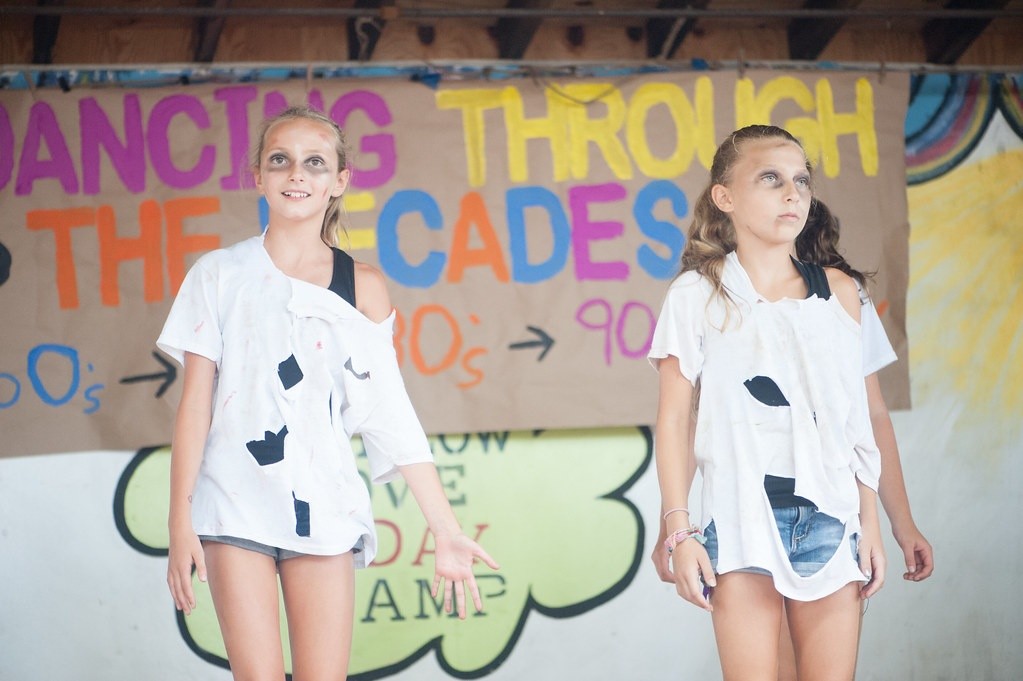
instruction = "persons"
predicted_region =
[646,124,885,681]
[651,194,934,681]
[154,107,502,681]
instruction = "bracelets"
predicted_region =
[663,508,690,521]
[664,524,707,556]
